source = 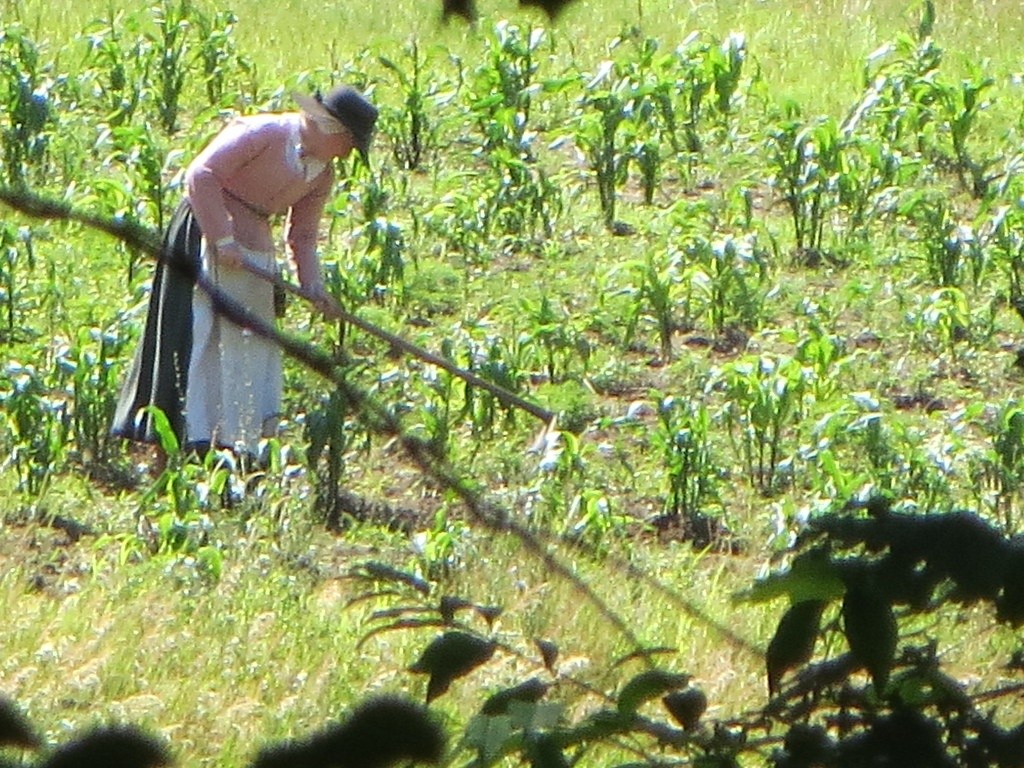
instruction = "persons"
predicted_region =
[109,80,380,483]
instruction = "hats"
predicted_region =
[316,85,377,169]
